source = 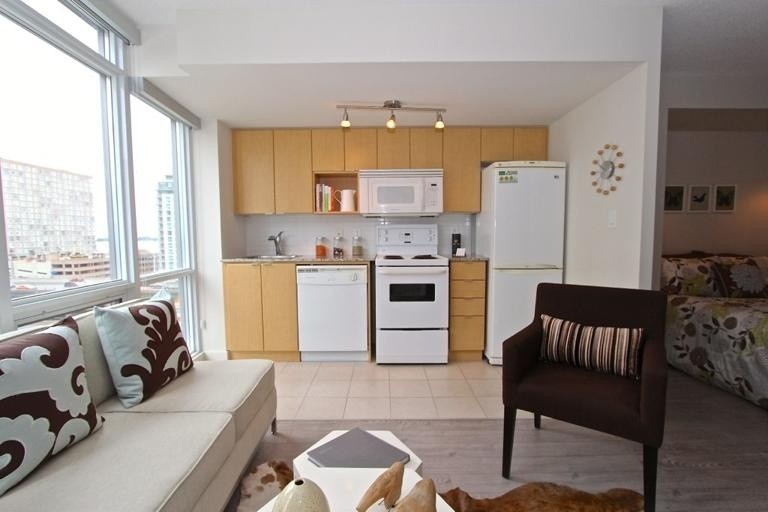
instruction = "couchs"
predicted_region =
[0,302,278,512]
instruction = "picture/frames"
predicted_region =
[713,184,738,214]
[686,183,713,214]
[662,183,686,214]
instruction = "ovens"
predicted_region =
[375,265,450,330]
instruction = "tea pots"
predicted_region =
[333,189,357,213]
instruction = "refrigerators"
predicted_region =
[475,161,571,365]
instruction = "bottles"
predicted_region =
[315,234,327,257]
[332,232,345,258]
[352,230,363,258]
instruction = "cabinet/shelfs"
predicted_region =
[450,261,487,352]
[234,126,314,215]
[444,126,481,212]
[482,126,551,162]
[314,127,378,174]
[223,259,299,353]
[377,125,443,169]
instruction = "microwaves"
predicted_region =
[357,167,444,218]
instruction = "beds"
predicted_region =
[660,250,767,415]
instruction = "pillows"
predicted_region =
[738,256,767,283]
[661,256,725,299]
[539,311,642,377]
[93,285,195,408]
[703,255,768,297]
[0,313,104,495]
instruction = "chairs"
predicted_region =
[502,281,667,510]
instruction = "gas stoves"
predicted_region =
[372,253,450,267]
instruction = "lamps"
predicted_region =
[335,102,449,130]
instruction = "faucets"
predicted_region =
[268,231,284,256]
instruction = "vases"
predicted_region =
[271,476,330,512]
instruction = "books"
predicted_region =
[306,426,411,469]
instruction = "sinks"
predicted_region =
[244,255,299,259]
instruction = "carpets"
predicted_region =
[236,459,644,512]
